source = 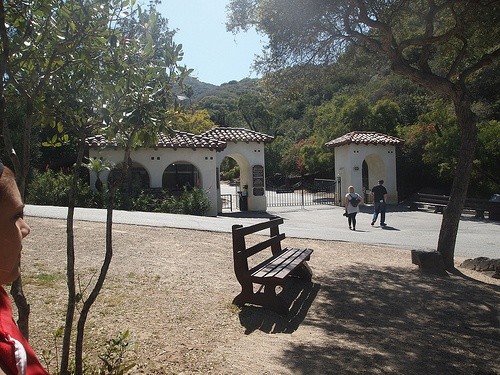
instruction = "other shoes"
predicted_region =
[380,223,386,225]
[371,221,375,225]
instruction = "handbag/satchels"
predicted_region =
[349,194,360,207]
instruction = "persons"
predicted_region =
[0,162,51,375]
[371,179,388,226]
[345,185,363,230]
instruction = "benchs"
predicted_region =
[411,193,449,216]
[463,198,500,223]
[231,217,313,315]
